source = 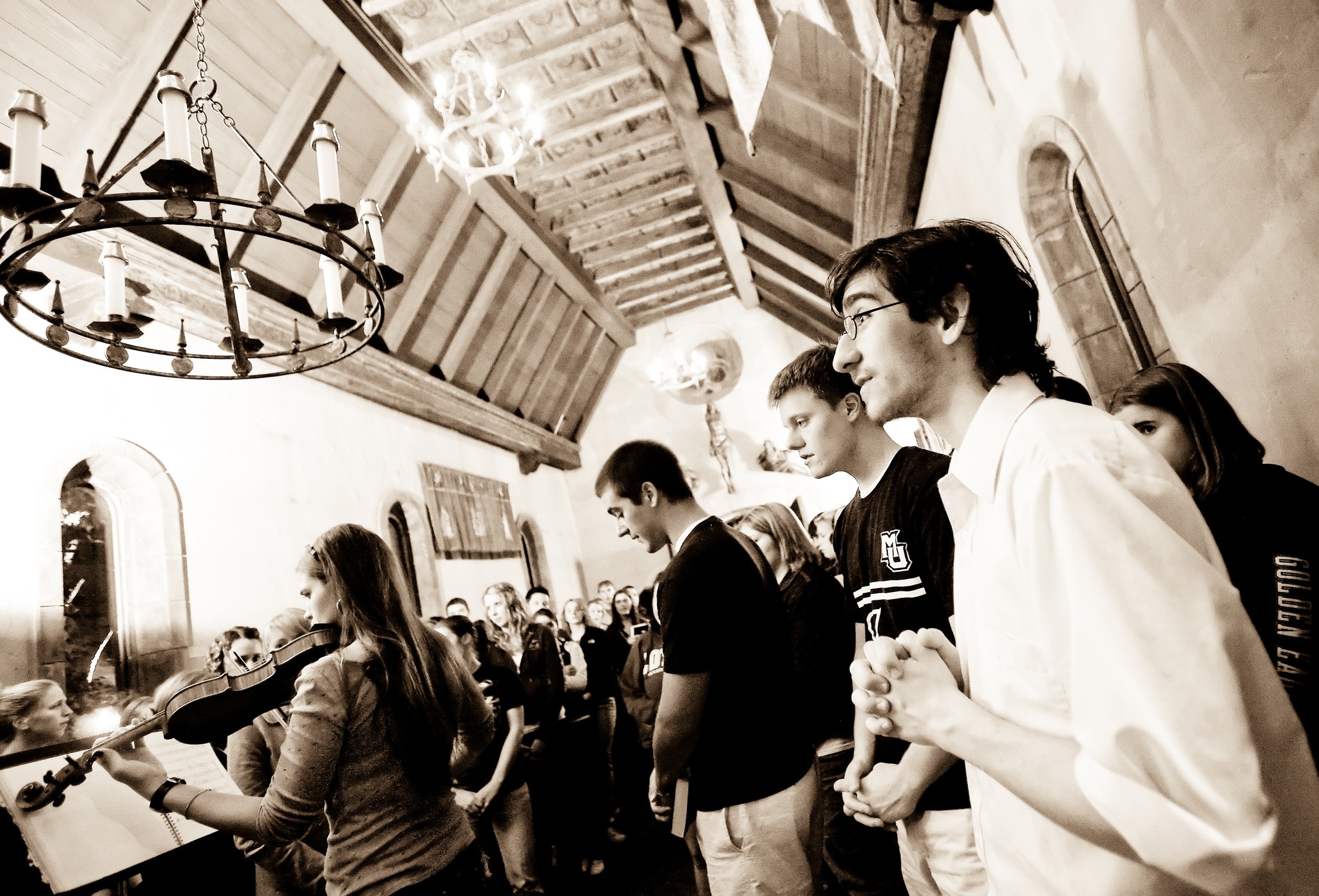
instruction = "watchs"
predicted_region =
[149,777,186,814]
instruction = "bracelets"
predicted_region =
[184,788,212,820]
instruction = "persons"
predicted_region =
[733,217,1319,896]
[0,606,328,896]
[417,569,697,896]
[95,522,490,896]
[595,440,822,896]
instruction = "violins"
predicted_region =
[15,629,337,813]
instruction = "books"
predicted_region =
[670,770,698,838]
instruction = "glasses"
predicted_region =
[837,302,901,342]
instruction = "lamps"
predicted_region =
[402,1,545,200]
[0,1,405,381]
